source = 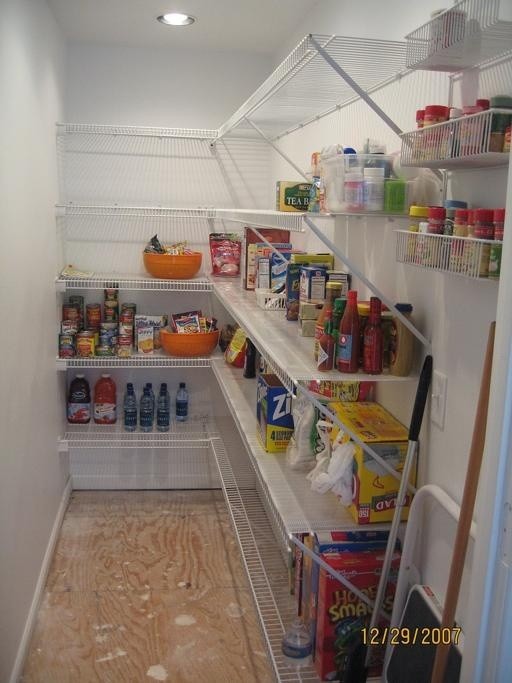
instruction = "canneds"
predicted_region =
[60,283,137,358]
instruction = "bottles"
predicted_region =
[405,199,505,280]
[338,145,404,215]
[280,616,313,675]
[412,95,512,162]
[242,339,256,378]
[123,378,190,432]
[311,281,413,378]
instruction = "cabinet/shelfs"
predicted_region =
[49,121,213,450]
[392,0,510,283]
[214,31,433,683]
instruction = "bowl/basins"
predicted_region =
[157,326,219,357]
[142,250,200,278]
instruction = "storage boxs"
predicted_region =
[319,153,396,214]
[255,368,418,527]
